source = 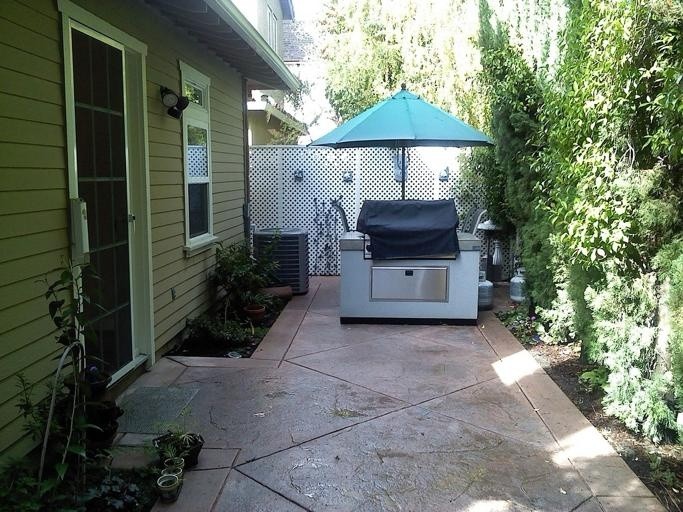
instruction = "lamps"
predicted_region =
[160,86,189,118]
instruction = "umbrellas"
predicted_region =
[306,83,497,202]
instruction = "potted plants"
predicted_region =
[141,404,206,502]
[212,225,283,327]
[12,251,127,451]
